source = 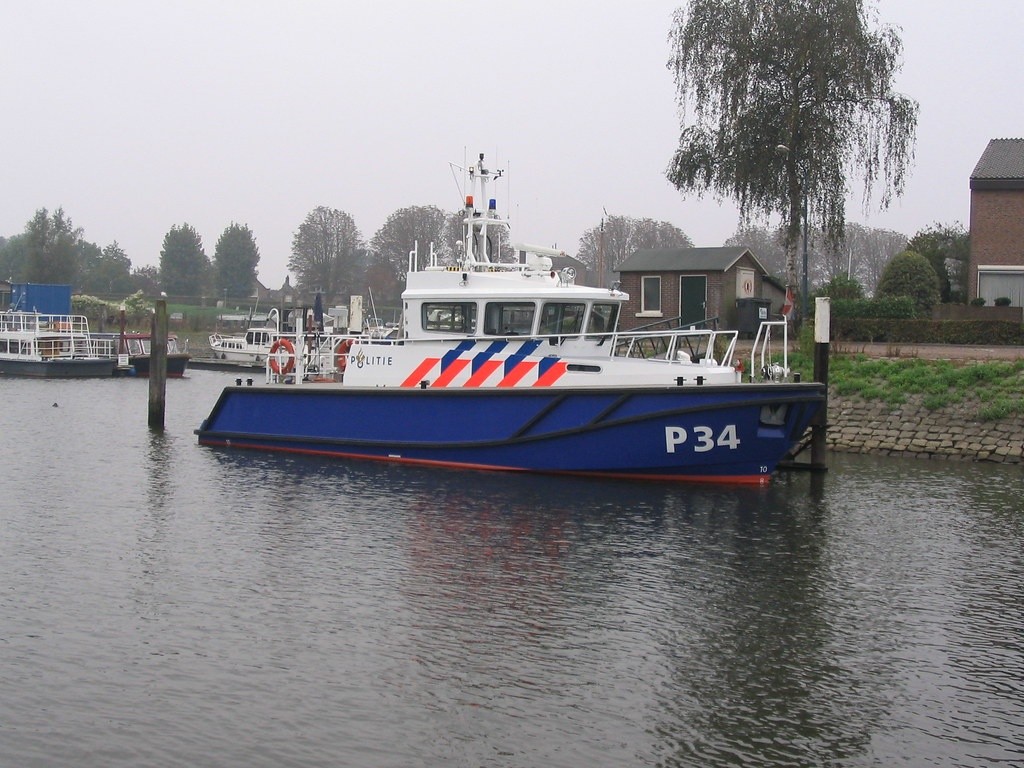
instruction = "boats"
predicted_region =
[205,291,342,374]
[195,155,827,488]
[0,278,191,378]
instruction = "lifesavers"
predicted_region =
[336,338,357,372]
[268,338,295,375]
